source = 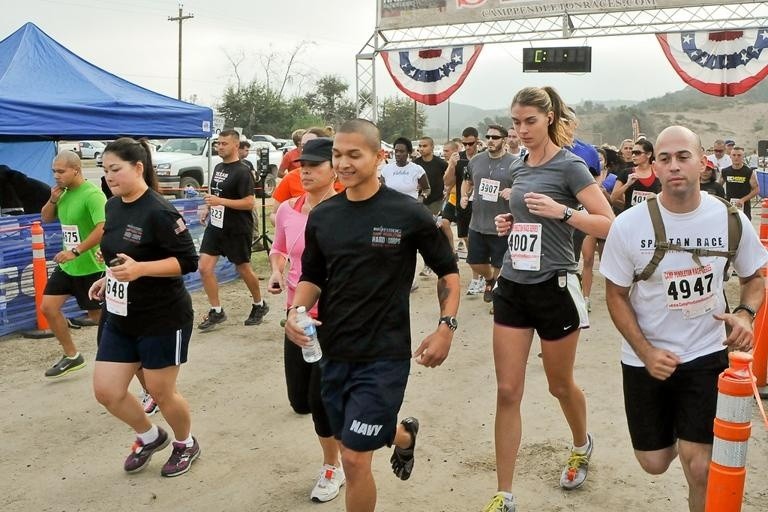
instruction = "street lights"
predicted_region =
[167,0,194,101]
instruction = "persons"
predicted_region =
[88,138,200,477]
[235,140,263,183]
[748,150,760,172]
[724,139,738,162]
[485,86,616,511]
[440,141,463,262]
[701,159,726,200]
[707,140,732,194]
[42,150,109,380]
[558,106,601,262]
[268,138,345,503]
[507,126,528,160]
[616,139,637,173]
[443,127,485,295]
[599,125,764,512]
[270,128,348,227]
[718,147,759,213]
[279,129,309,176]
[581,146,625,310]
[197,130,268,330]
[416,134,448,277]
[380,136,431,204]
[283,119,461,512]
[699,145,718,161]
[461,125,527,314]
[611,139,663,210]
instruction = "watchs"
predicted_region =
[420,192,430,199]
[437,315,459,331]
[561,203,575,224]
[734,304,758,320]
[71,247,79,253]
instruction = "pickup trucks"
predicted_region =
[151,134,284,198]
[58,140,106,159]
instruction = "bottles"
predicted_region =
[294,306,322,363]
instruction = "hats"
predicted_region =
[558,106,576,122]
[292,137,334,163]
[724,140,735,145]
[706,161,716,170]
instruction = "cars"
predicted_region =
[434,145,443,157]
[381,141,394,159]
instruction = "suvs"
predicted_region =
[278,141,297,154]
[251,134,286,149]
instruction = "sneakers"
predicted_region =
[123,426,171,475]
[197,308,228,331]
[244,299,270,327]
[389,417,419,482]
[418,264,434,276]
[583,297,592,312]
[467,279,478,296]
[310,461,347,504]
[483,493,517,512]
[143,398,160,416]
[456,238,465,251]
[44,351,86,379]
[409,279,419,292]
[141,392,151,406]
[454,253,460,263]
[560,431,594,490]
[160,436,201,478]
[484,280,496,303]
[474,274,485,294]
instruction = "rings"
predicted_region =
[531,202,536,209]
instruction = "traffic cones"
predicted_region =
[758,196,767,259]
[22,220,55,339]
[703,351,756,512]
[751,268,767,406]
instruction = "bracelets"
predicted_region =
[48,199,58,204]
[285,305,298,317]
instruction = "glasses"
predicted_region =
[704,167,711,171]
[485,134,504,140]
[713,149,724,153]
[462,139,480,146]
[630,150,648,157]
